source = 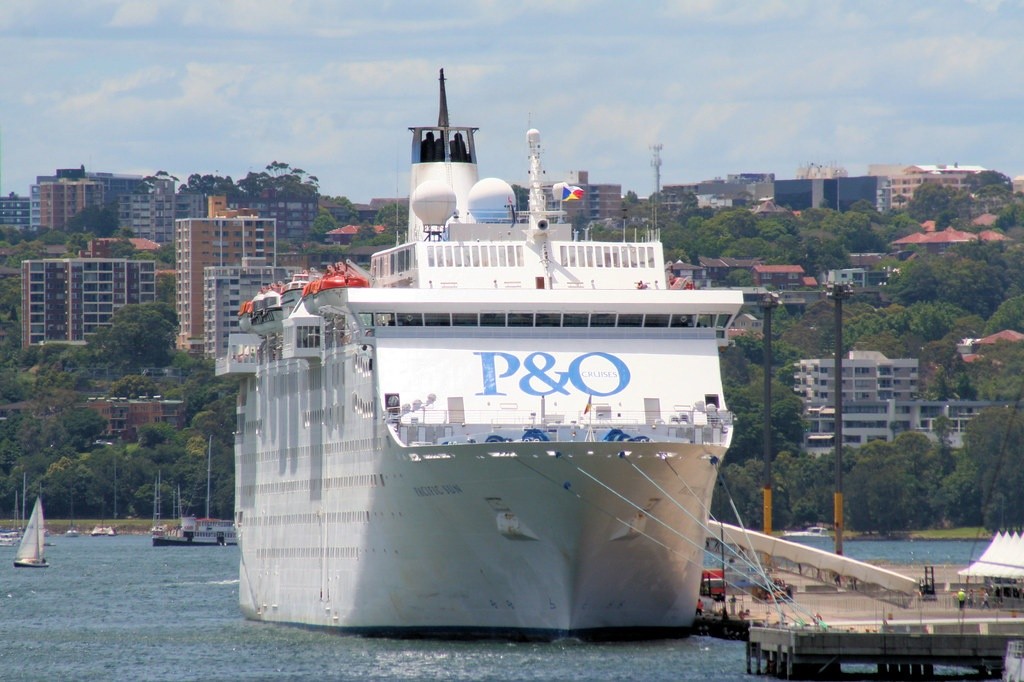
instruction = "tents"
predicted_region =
[957,529,1024,600]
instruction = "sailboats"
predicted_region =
[0,434,240,569]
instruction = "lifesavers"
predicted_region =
[738,611,745,619]
[718,609,724,615]
[813,615,823,624]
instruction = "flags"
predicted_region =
[584,396,591,414]
[563,186,584,201]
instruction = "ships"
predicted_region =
[235,63,747,642]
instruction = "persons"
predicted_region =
[696,599,704,616]
[968,588,974,608]
[453,133,469,161]
[982,589,991,610]
[728,594,737,614]
[995,587,1003,603]
[957,589,965,609]
[421,131,437,162]
